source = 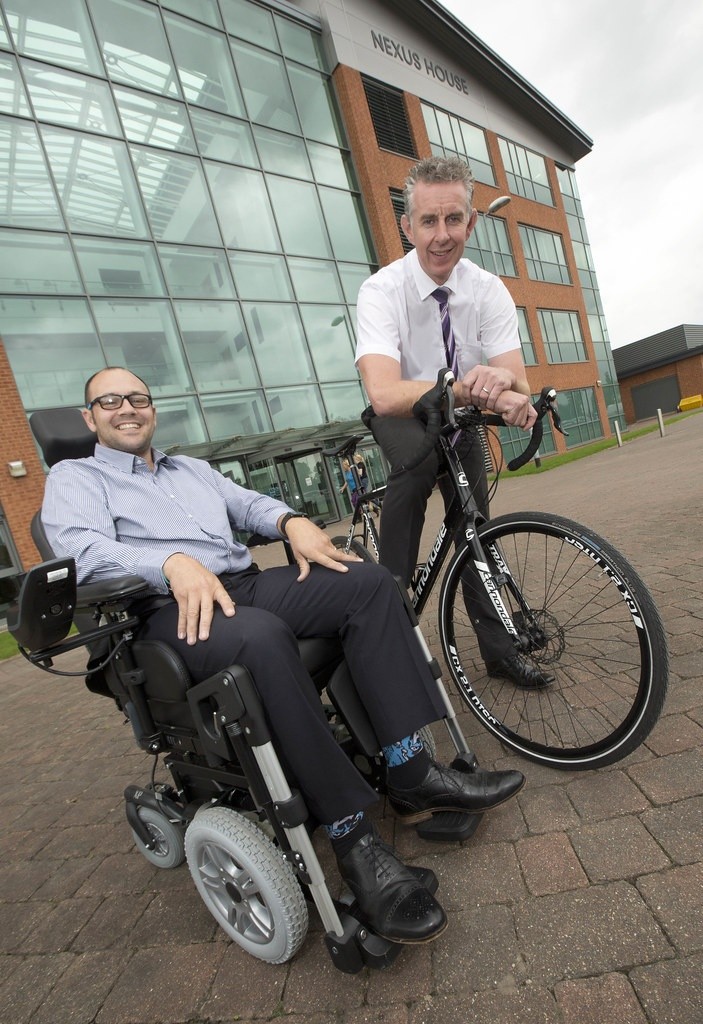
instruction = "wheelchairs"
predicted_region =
[3,508,486,977]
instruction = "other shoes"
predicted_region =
[379,501,383,509]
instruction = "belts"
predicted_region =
[140,597,175,612]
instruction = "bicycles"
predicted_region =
[319,369,668,772]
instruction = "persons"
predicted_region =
[40,367,524,945]
[354,154,556,690]
[338,455,383,523]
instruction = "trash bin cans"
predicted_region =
[679,394,702,411]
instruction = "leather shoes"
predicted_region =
[385,739,526,826]
[336,821,450,946]
[484,654,558,689]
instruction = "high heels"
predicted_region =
[357,514,362,523]
[373,506,380,517]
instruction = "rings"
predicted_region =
[483,388,490,395]
[334,548,337,551]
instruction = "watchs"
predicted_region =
[281,511,310,539]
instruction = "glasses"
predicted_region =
[86,393,153,410]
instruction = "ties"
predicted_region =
[431,288,459,383]
